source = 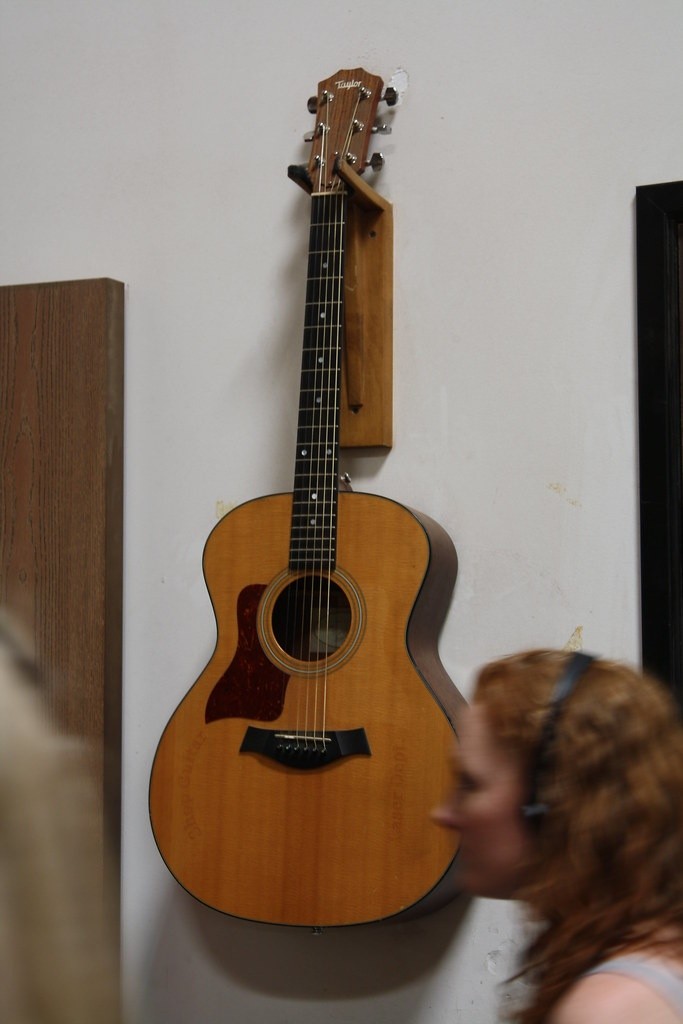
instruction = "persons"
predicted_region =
[1,609,120,1024]
[427,649,683,1024]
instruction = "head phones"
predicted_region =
[519,652,594,829]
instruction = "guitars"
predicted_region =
[147,68,500,932]
